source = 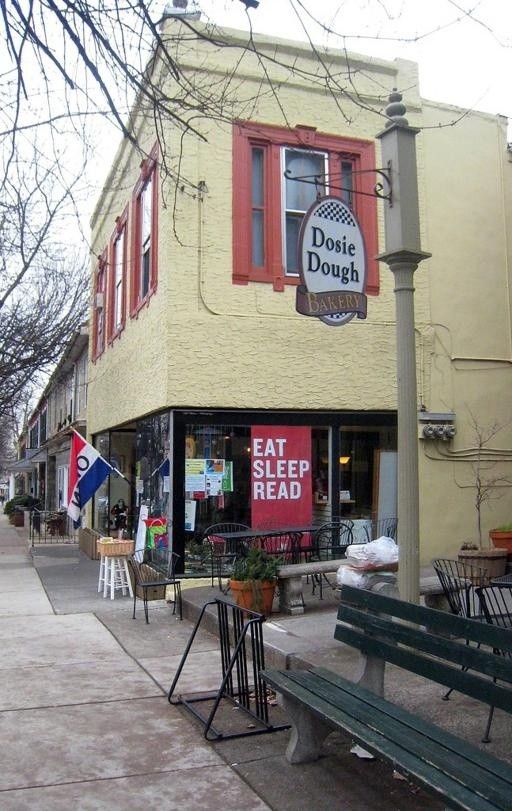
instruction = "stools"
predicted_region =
[96,555,135,600]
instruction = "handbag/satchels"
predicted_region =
[146,519,167,550]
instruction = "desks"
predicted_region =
[313,500,356,520]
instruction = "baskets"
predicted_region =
[96,539,134,556]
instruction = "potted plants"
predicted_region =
[489,522,512,561]
[230,548,277,618]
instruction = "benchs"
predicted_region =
[257,585,512,811]
[277,558,348,615]
[373,577,472,614]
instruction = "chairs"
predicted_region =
[432,558,487,674]
[202,515,397,601]
[490,574,512,585]
[127,547,182,624]
[442,582,512,743]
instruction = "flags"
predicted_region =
[65,428,115,525]
[159,451,170,484]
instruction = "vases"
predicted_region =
[458,548,508,585]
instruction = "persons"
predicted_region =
[316,469,328,496]
[206,460,214,473]
[114,499,129,541]
